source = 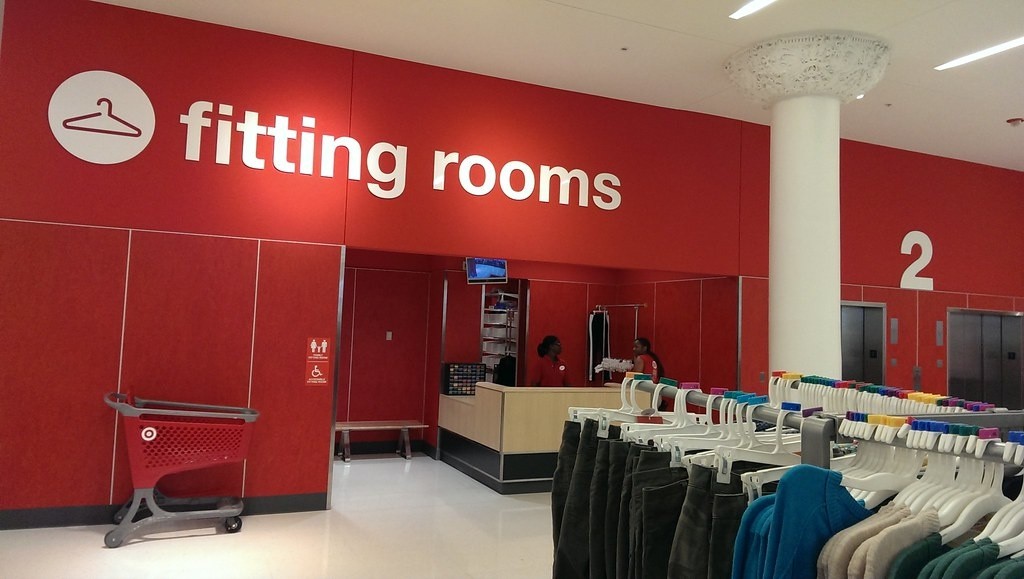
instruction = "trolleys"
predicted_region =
[102,391,260,549]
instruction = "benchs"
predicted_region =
[335,419,429,461]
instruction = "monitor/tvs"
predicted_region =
[466,256,508,284]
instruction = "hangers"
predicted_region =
[567,371,1024,562]
[591,304,607,315]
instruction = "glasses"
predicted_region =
[556,342,560,346]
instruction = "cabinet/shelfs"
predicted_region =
[483,293,518,383]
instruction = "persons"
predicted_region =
[531,336,583,387]
[630,338,664,384]
